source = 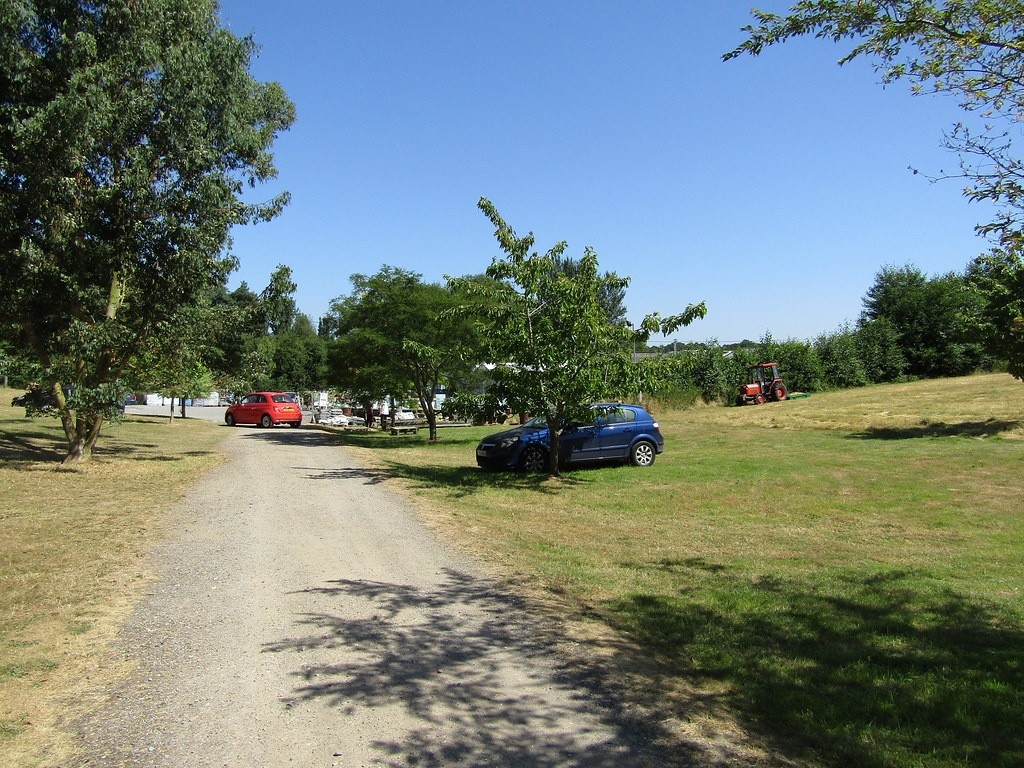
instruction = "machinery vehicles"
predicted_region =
[736,361,787,406]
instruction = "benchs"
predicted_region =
[390,426,417,435]
[345,427,369,433]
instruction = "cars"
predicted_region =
[124,393,137,406]
[11,386,72,410]
[224,391,303,429]
[475,403,666,474]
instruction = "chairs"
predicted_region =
[319,411,433,428]
